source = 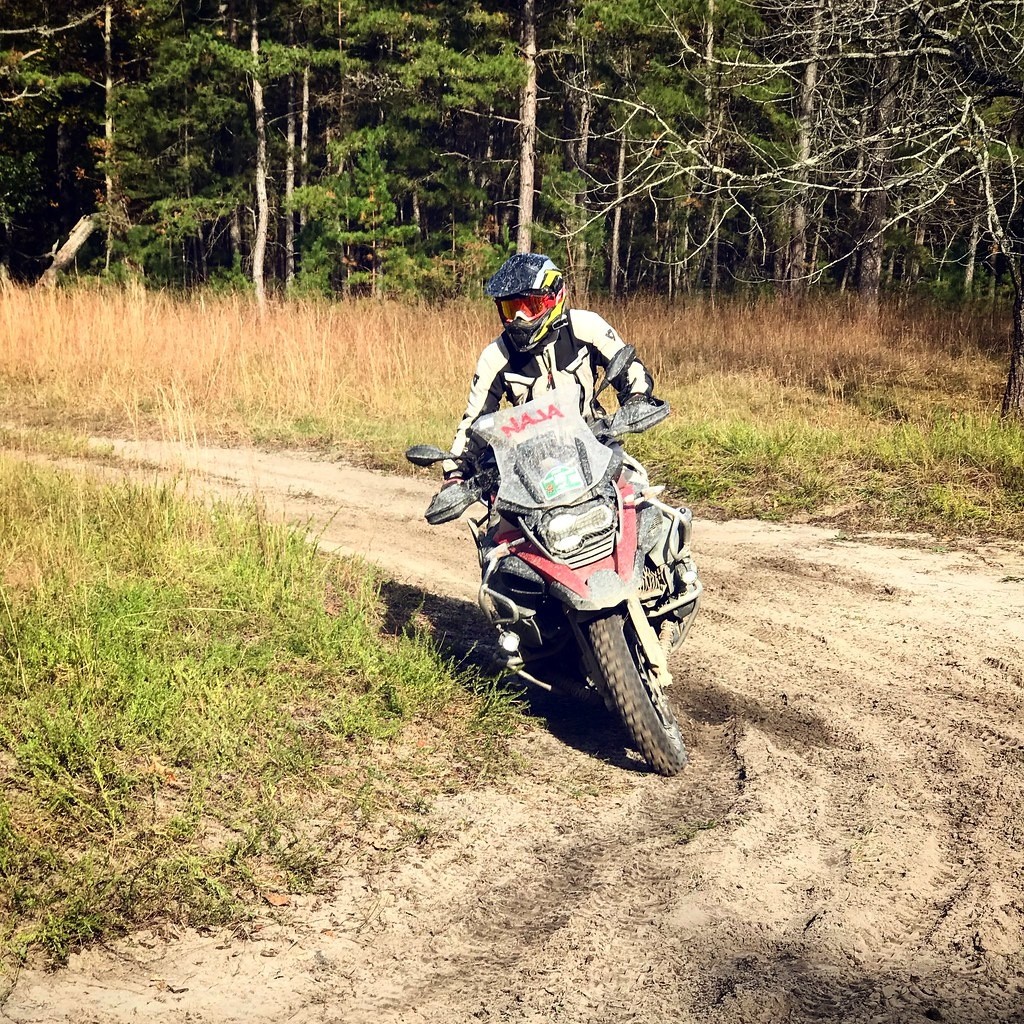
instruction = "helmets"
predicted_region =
[486,252,565,354]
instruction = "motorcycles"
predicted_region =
[407,344,703,776]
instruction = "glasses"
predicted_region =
[496,296,546,323]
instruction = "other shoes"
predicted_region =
[663,559,695,618]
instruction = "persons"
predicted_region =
[442,253,656,554]
